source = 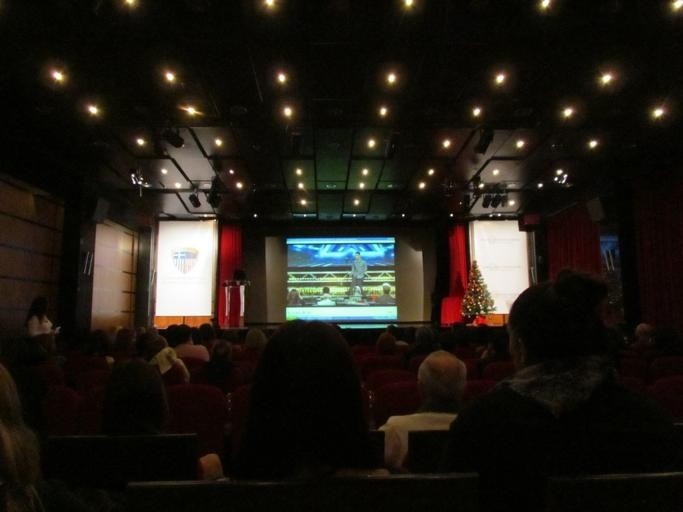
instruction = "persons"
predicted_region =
[352,318,508,366]
[442,267,681,479]
[68,321,226,385]
[287,251,395,307]
[97,357,222,480]
[232,329,267,366]
[0,349,85,512]
[375,349,467,471]
[26,296,59,348]
[226,319,391,476]
